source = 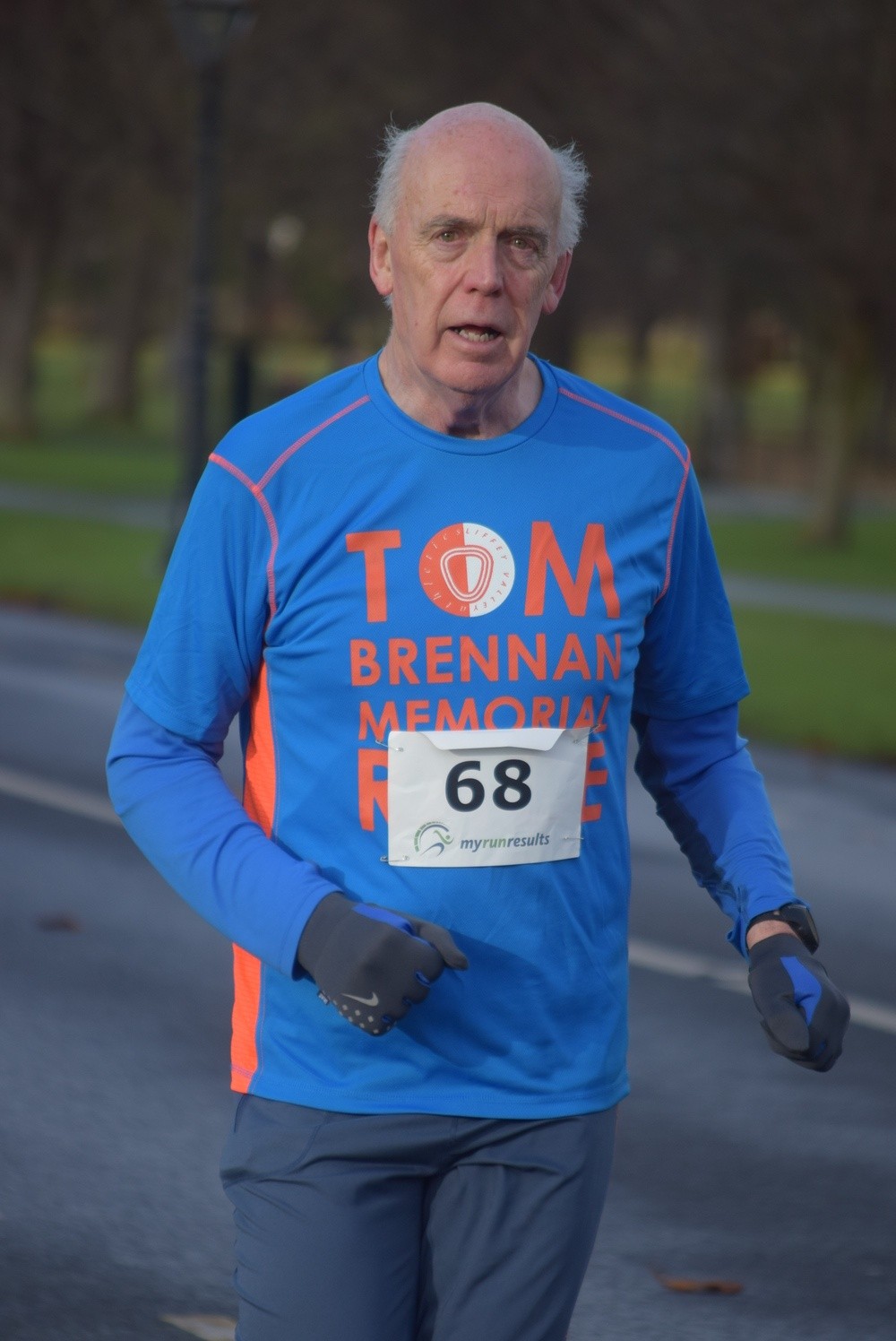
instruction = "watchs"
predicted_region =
[746,903,820,955]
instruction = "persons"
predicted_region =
[104,101,850,1341]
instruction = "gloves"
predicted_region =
[298,892,468,1038]
[748,932,851,1072]
